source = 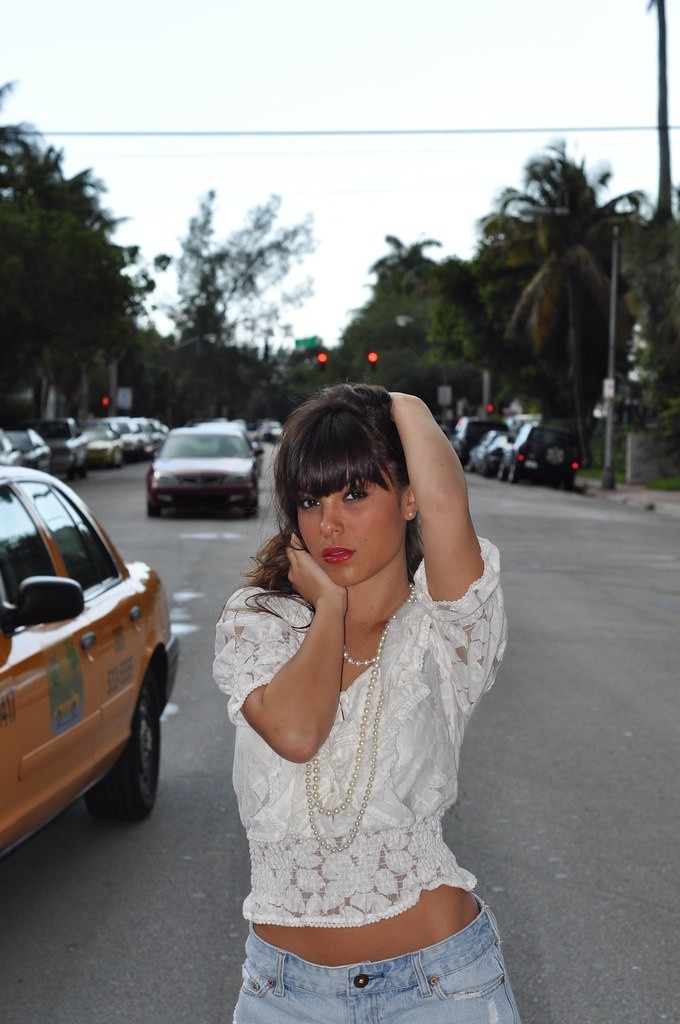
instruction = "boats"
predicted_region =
[366,347,380,369]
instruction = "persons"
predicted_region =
[212,383,527,1024]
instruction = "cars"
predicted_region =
[81,415,292,471]
[448,415,584,490]
[0,426,54,472]
[145,427,264,520]
[0,464,184,864]
[21,417,92,482]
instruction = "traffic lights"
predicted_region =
[315,351,329,372]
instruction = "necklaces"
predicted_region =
[305,584,417,854]
